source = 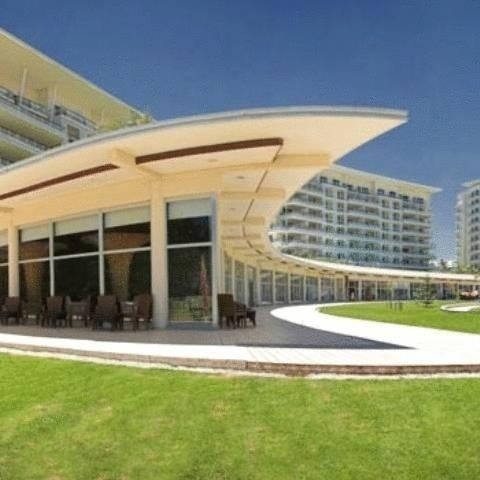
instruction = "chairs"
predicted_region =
[1,293,256,331]
